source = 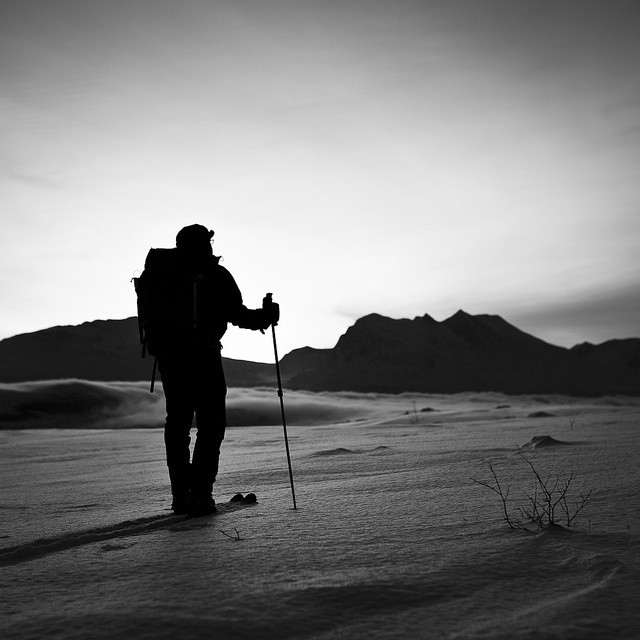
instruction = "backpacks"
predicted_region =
[133,249,221,392]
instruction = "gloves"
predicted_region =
[258,302,279,329]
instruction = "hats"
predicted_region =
[176,224,214,245]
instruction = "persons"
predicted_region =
[158,224,280,512]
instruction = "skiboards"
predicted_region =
[171,492,258,517]
